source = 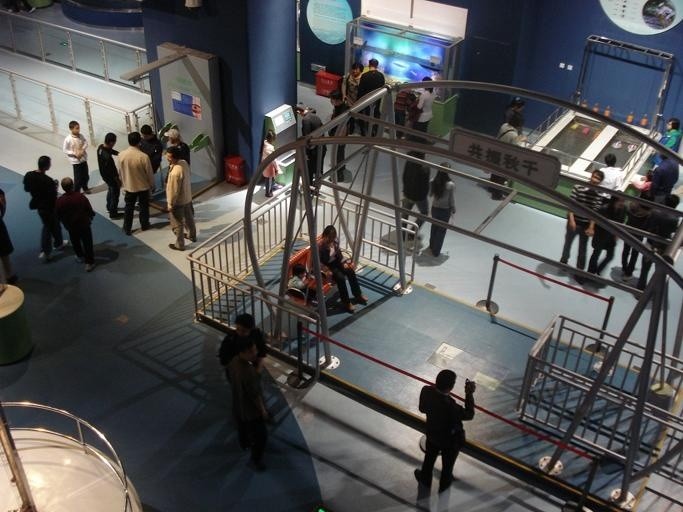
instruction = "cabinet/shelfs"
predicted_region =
[344,15,464,99]
[427,94,458,138]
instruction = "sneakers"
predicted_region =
[556,261,648,300]
[343,295,368,315]
[37,188,198,273]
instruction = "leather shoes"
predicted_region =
[413,469,421,482]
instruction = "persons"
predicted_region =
[260,130,282,197]
[218,312,267,451]
[284,224,368,313]
[288,58,437,184]
[228,340,270,474]
[401,151,455,258]
[414,369,476,493]
[559,117,683,300]
[0,120,197,287]
[489,96,528,200]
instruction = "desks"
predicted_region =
[0,284,33,366]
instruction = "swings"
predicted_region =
[284,144,371,311]
